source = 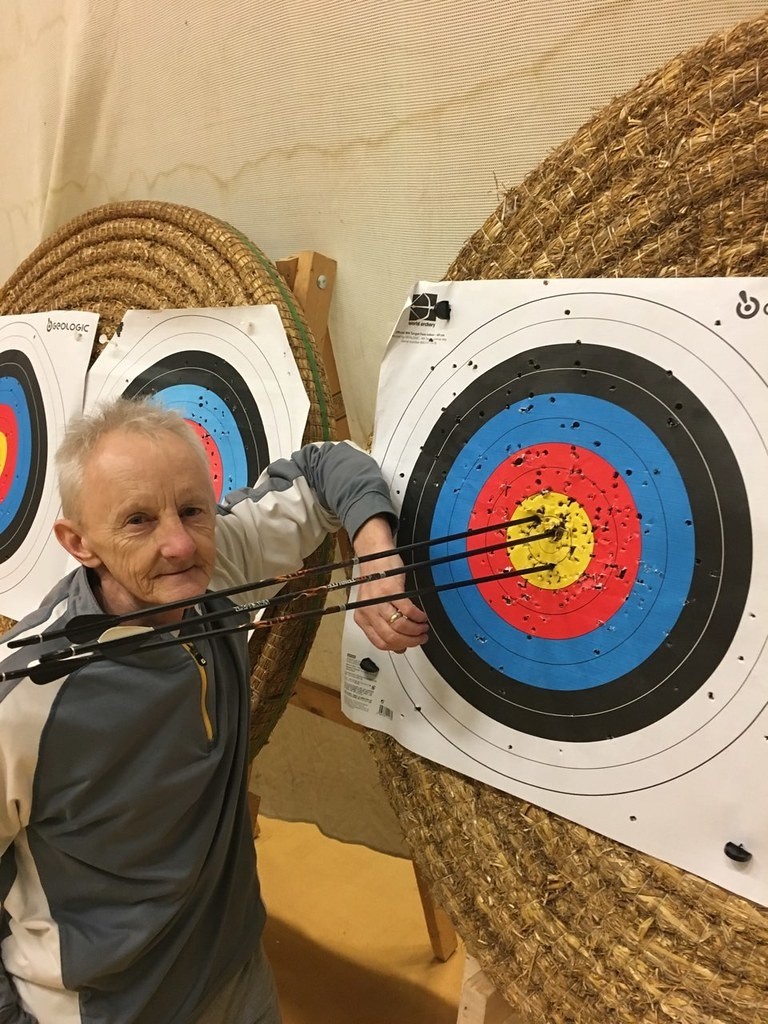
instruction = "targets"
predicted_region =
[86,304,313,515]
[0,309,102,624]
[341,276,768,913]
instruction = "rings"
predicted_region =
[388,612,402,625]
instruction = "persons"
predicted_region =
[0,394,430,1024]
